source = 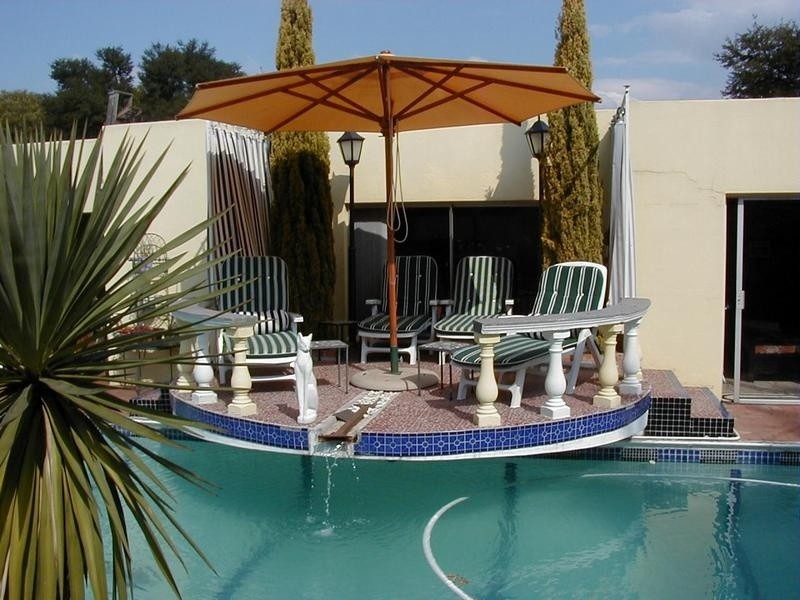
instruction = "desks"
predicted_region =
[318,320,359,364]
[418,340,474,401]
[308,340,348,393]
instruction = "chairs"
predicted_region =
[434,256,514,365]
[357,255,439,365]
[450,261,607,408]
[214,255,303,383]
